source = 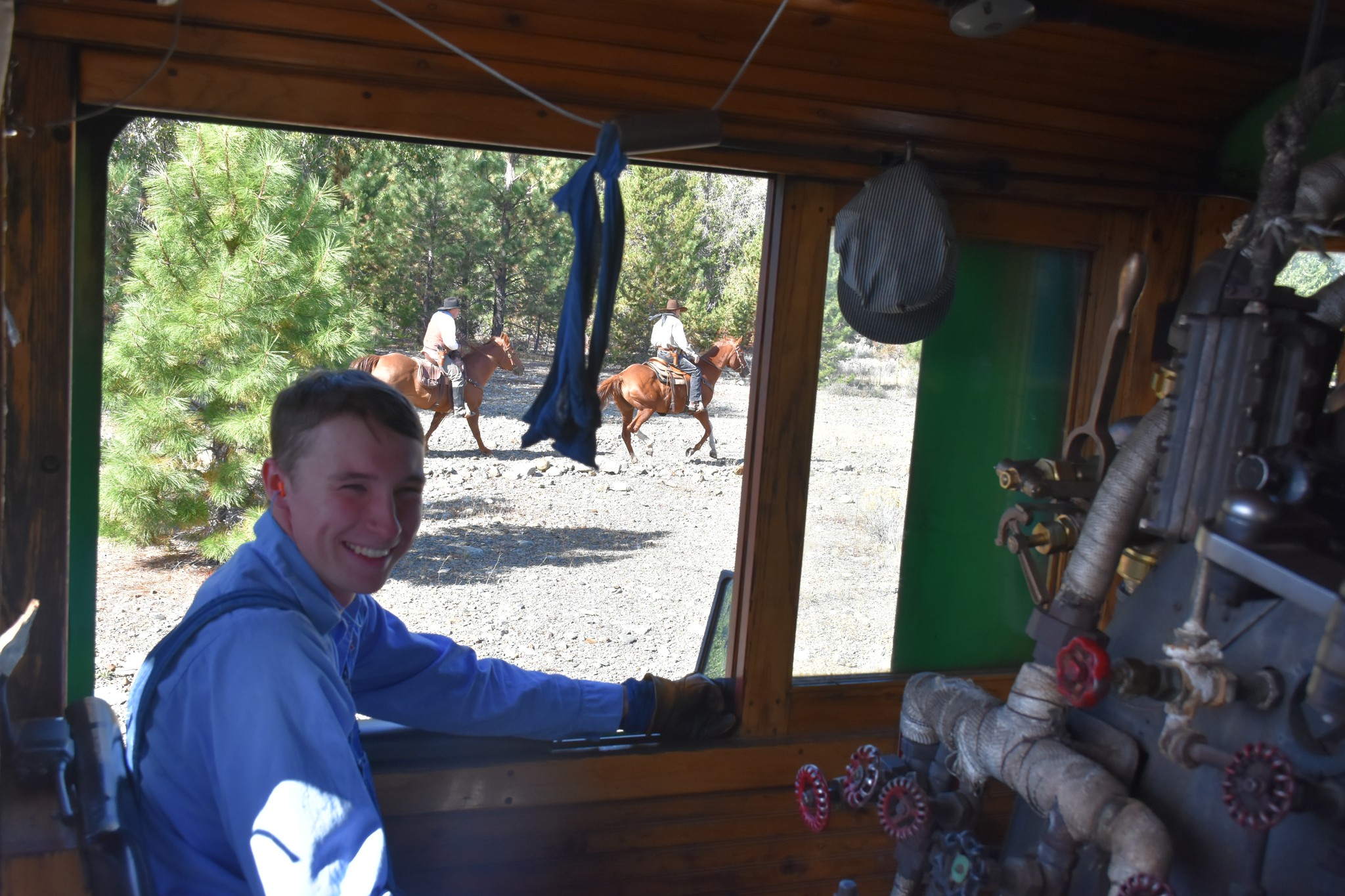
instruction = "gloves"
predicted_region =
[645,669,736,744]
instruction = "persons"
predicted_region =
[422,297,476,419]
[128,369,735,896]
[651,299,708,411]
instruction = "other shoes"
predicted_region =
[689,402,707,411]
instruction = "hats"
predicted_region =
[437,297,465,310]
[831,154,956,346]
[659,300,688,312]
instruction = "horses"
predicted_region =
[596,329,752,465]
[346,329,526,457]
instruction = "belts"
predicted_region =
[657,347,671,352]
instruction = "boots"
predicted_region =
[452,386,476,419]
